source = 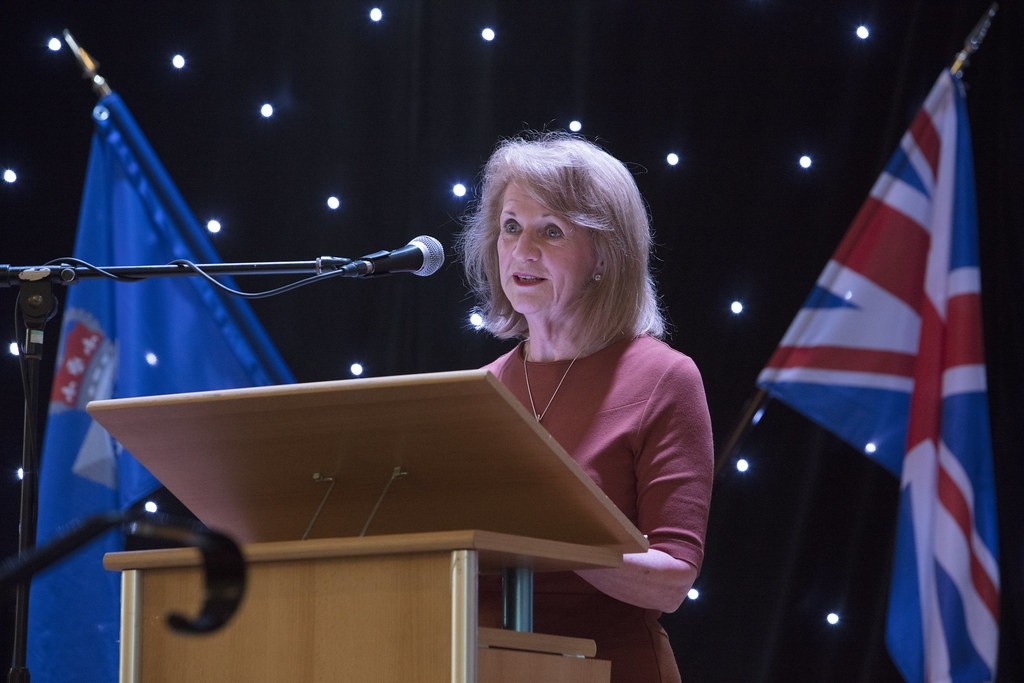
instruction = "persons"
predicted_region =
[462,134,714,683]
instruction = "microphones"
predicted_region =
[339,235,445,277]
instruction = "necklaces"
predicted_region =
[525,335,598,423]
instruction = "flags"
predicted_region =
[755,68,1003,683]
[27,96,297,683]
